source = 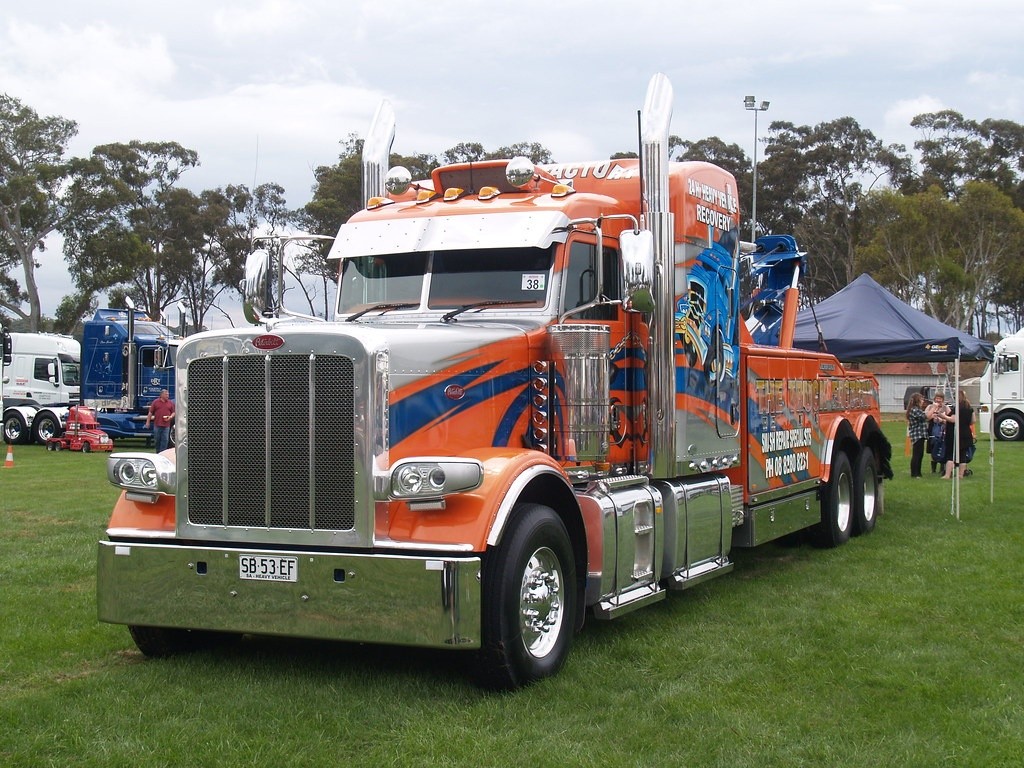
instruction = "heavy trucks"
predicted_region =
[4,331,82,447]
[45,406,115,453]
[80,307,184,449]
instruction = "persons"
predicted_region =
[143,389,175,453]
[936,391,976,480]
[925,393,950,476]
[906,393,938,478]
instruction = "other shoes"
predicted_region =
[931,471,937,475]
[959,475,963,479]
[963,468,973,476]
[940,475,950,479]
[937,469,945,476]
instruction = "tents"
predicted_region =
[796,273,995,520]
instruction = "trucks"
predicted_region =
[978,326,1024,442]
[0,322,12,442]
[94,70,896,693]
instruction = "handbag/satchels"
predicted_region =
[932,428,946,463]
[965,437,977,463]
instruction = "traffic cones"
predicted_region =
[2,444,15,469]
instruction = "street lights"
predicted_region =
[743,93,771,243]
